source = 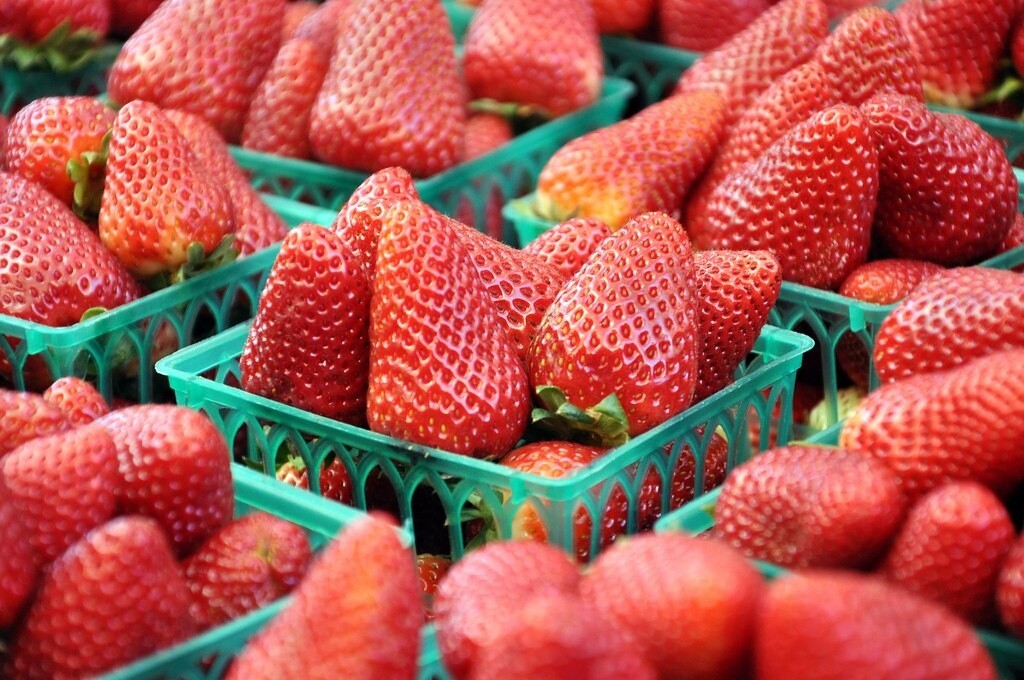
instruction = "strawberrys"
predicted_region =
[1,0,1024,680]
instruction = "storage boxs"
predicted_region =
[0,0,1024,680]
